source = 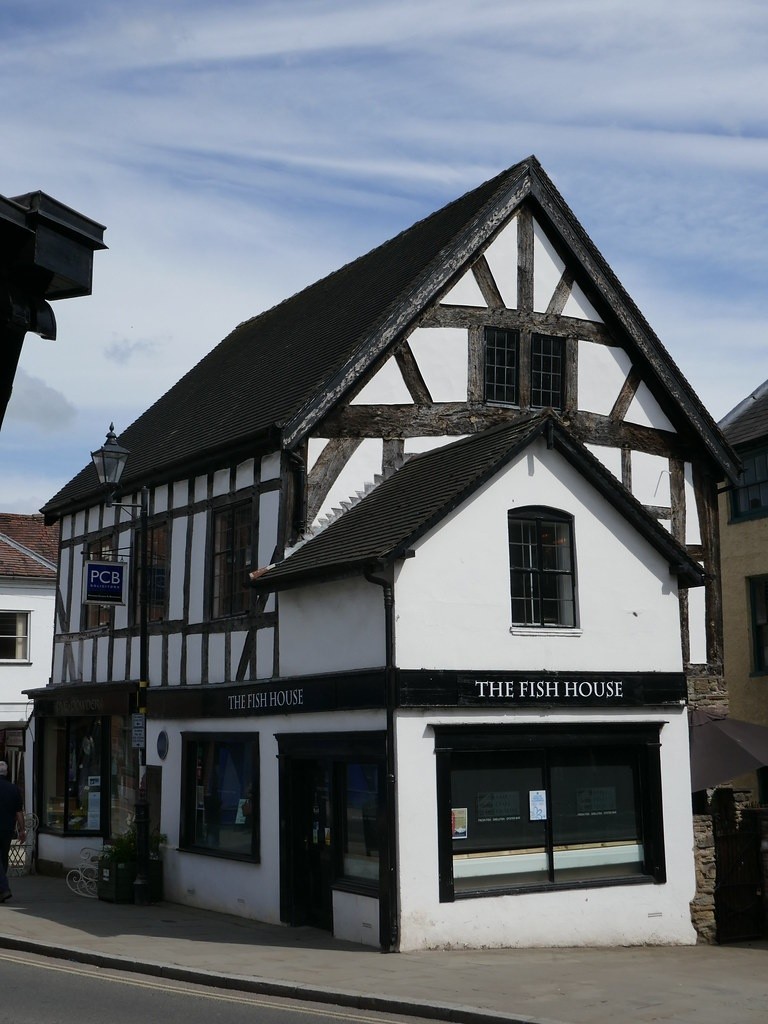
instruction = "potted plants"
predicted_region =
[99,819,165,904]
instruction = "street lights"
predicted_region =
[92,424,152,903]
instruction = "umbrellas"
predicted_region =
[688,705,768,792]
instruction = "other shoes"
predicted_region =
[0,889,12,902]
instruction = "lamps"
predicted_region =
[90,422,148,523]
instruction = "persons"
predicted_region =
[0,761,28,903]
